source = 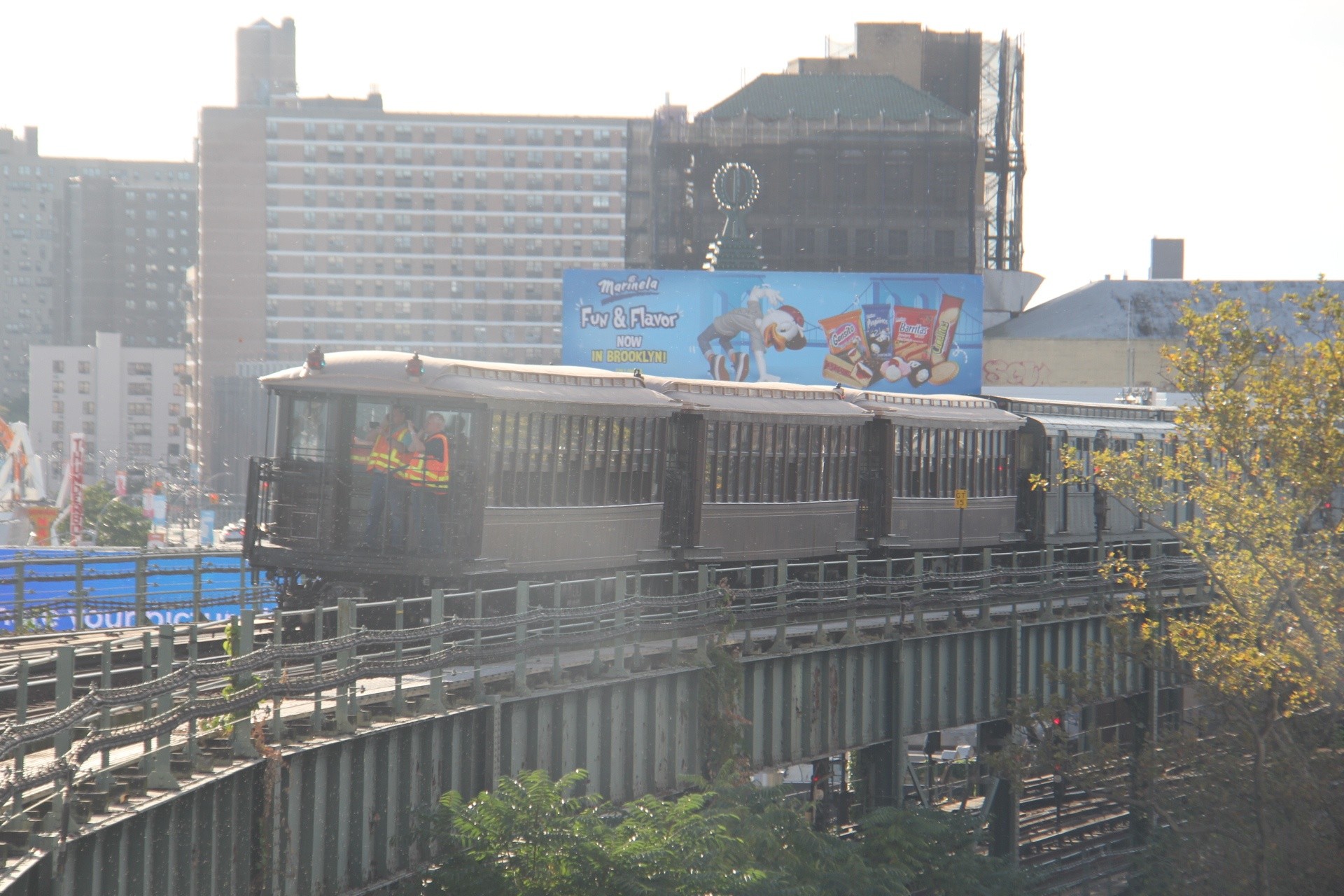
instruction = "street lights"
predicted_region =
[1055,763,1063,784]
[811,774,825,832]
[87,449,119,485]
[1322,493,1331,512]
[128,452,234,546]
[1093,437,1104,516]
[41,449,63,500]
[1052,711,1061,736]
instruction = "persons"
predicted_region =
[402,412,454,556]
[362,401,415,545]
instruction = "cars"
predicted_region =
[219,524,244,545]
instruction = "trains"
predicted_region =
[234,344,1343,659]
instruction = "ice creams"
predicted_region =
[818,292,965,388]
